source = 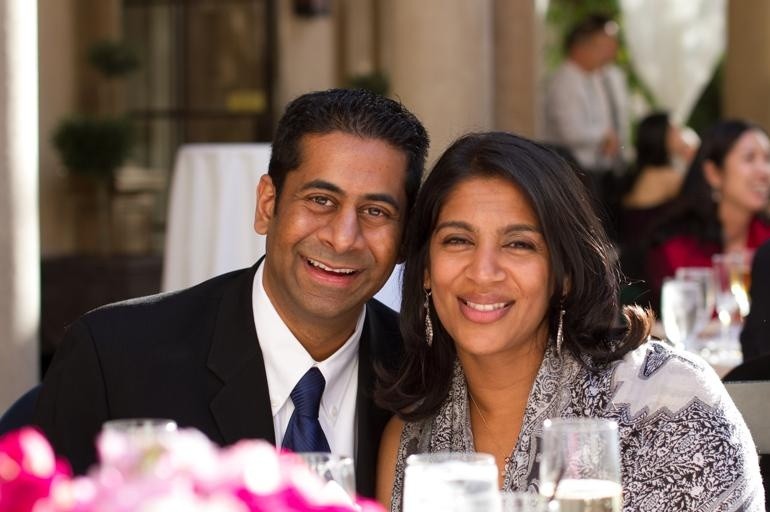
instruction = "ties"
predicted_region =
[601,78,623,161]
[280,367,331,453]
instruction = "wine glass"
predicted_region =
[657,252,747,360]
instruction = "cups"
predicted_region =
[541,414,626,512]
[401,448,503,512]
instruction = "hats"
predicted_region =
[561,9,614,58]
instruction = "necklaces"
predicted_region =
[468,389,510,463]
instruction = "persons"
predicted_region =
[372,133,766,512]
[594,112,768,380]
[548,13,633,182]
[35,87,430,501]
